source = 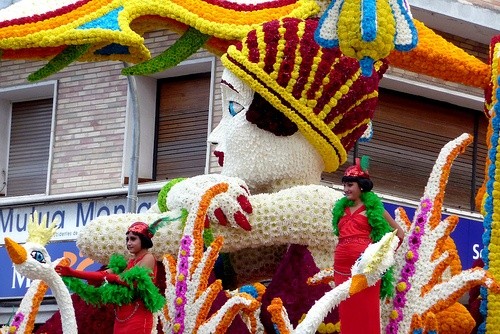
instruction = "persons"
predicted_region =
[332,164,404,334]
[54,222,157,334]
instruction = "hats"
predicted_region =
[343,155,370,179]
[127,215,182,239]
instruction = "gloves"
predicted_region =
[54,264,77,277]
